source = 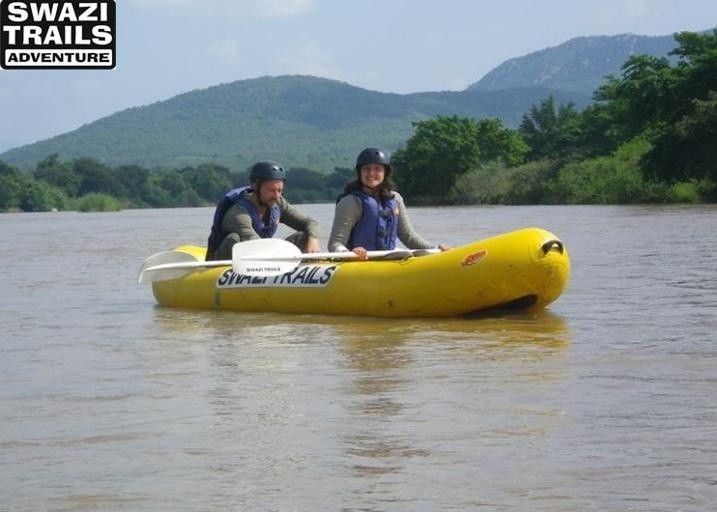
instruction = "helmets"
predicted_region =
[248,160,287,180]
[354,147,392,170]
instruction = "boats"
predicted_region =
[152,226,570,319]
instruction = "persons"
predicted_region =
[326,145,451,262]
[203,159,323,263]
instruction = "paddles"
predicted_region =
[137,238,442,287]
